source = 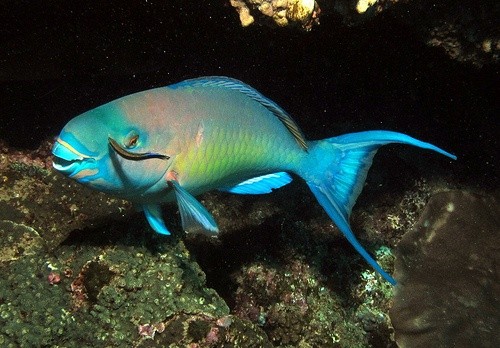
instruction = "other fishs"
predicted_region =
[52,75,456,287]
